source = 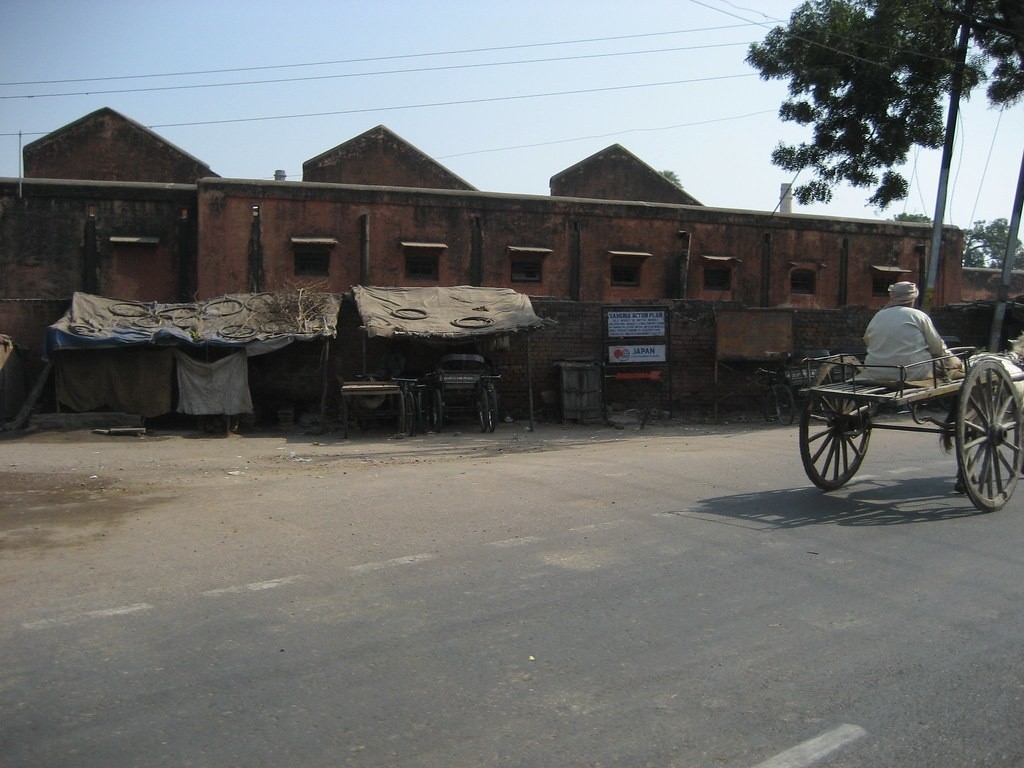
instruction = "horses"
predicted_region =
[937,332,1023,495]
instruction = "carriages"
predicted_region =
[795,330,1024,512]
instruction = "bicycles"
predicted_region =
[754,367,796,427]
[390,373,503,436]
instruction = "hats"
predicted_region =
[887,281,919,305]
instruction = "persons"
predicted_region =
[865,281,963,381]
